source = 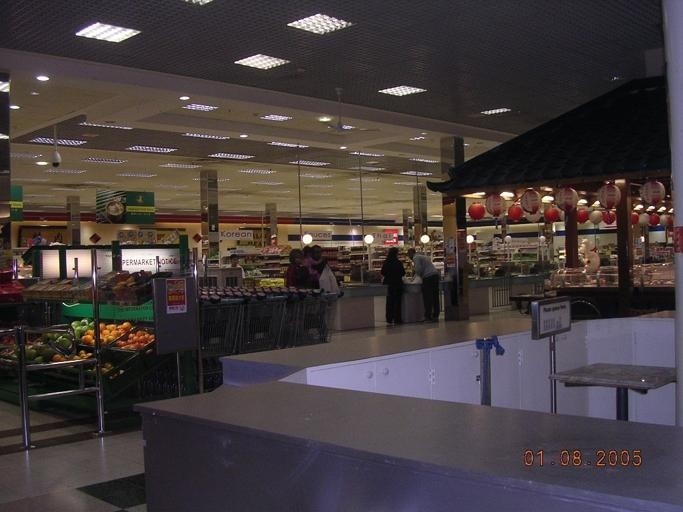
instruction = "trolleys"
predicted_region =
[196,286,344,357]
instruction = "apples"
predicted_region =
[25,319,155,376]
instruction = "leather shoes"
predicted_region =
[416,317,438,323]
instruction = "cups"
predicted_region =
[105,200,125,223]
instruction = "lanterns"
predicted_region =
[466,177,673,229]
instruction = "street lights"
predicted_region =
[504,235,512,262]
[539,236,546,261]
[302,232,314,248]
[466,233,474,263]
[365,234,374,270]
[421,234,430,257]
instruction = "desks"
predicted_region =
[549,361,676,420]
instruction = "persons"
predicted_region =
[312,244,325,291]
[286,248,310,288]
[381,246,405,325]
[407,246,440,324]
[224,254,245,278]
[302,244,322,282]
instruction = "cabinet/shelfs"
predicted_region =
[522,331,588,416]
[310,351,431,403]
[1,244,184,417]
[590,328,674,425]
[185,244,683,287]
[433,336,522,412]
